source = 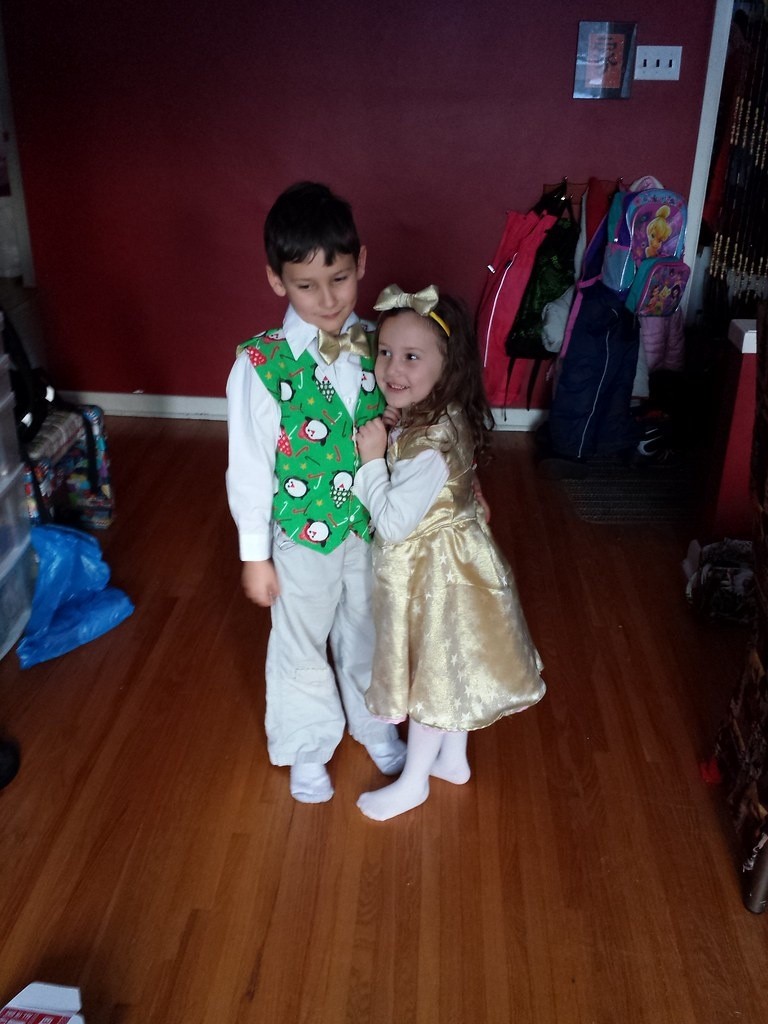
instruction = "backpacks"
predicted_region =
[599,176,688,294]
[505,200,580,360]
[0,306,64,444]
[624,253,690,317]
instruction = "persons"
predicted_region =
[225,181,409,803]
[349,283,546,821]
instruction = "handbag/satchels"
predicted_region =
[15,523,136,669]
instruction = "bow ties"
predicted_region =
[319,323,371,364]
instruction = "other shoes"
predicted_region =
[0,742,20,791]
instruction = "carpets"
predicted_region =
[563,461,690,525]
